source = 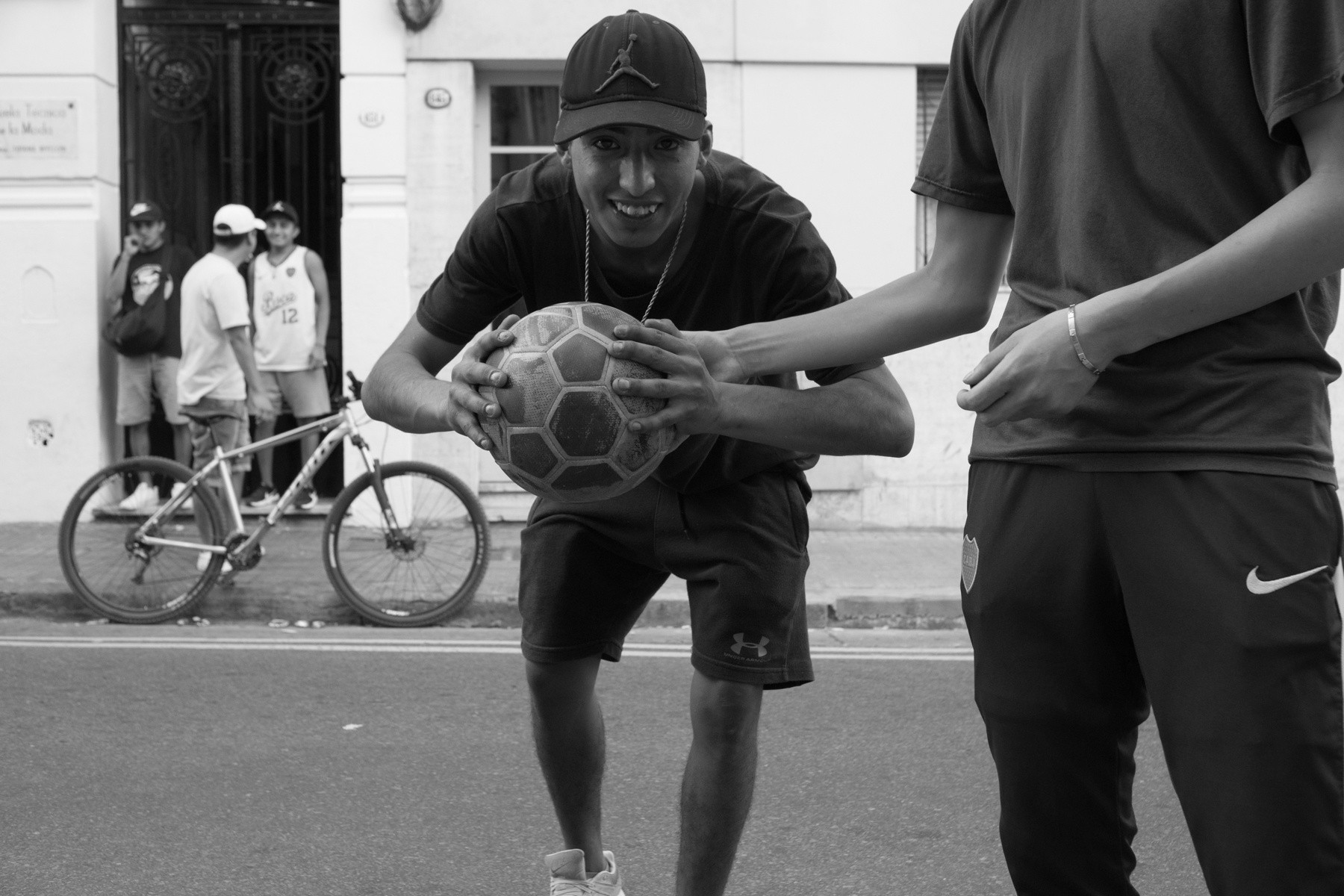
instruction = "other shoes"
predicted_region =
[246,484,281,506]
[545,849,626,896]
[118,481,161,510]
[171,487,192,510]
[294,486,318,509]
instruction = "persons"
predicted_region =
[106,199,197,510]
[177,202,273,574]
[643,0,1344,896]
[359,10,915,895]
[248,200,331,508]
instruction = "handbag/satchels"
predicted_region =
[101,285,166,358]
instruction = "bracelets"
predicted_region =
[1067,304,1102,376]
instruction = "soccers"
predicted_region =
[477,300,676,505]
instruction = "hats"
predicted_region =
[551,9,707,144]
[263,200,298,226]
[126,201,163,223]
[213,204,267,236]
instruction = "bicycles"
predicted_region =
[57,369,491,628]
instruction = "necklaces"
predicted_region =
[584,205,688,322]
